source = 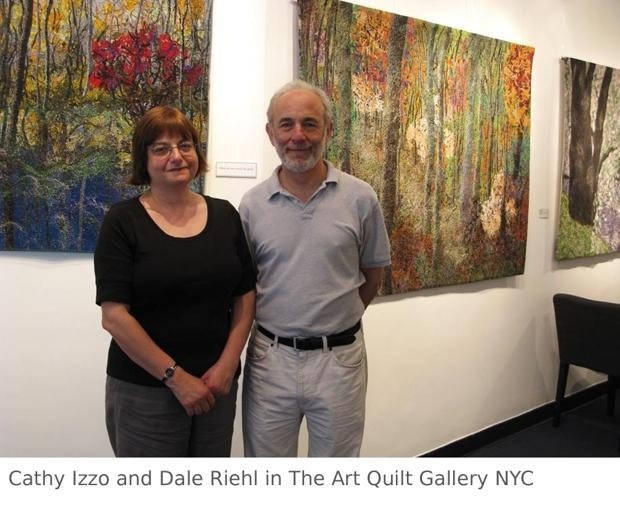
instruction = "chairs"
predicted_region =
[553,292,620,427]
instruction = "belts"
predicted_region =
[257,320,360,350]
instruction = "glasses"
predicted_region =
[147,142,197,160]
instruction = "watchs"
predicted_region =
[161,362,179,383]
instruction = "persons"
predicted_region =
[236,81,393,457]
[93,106,257,457]
